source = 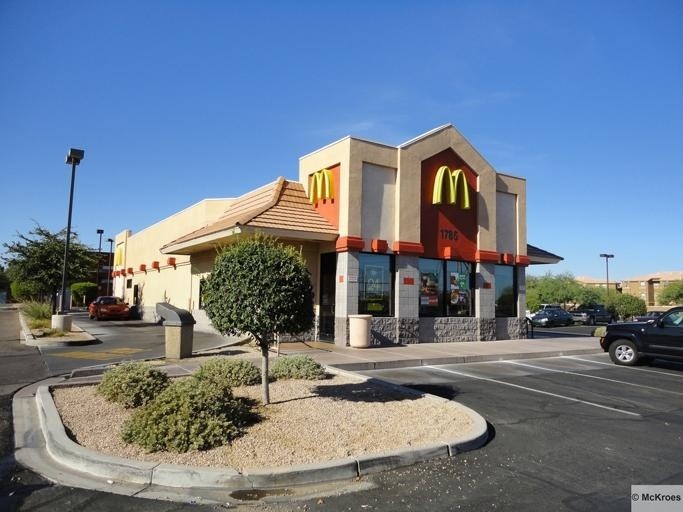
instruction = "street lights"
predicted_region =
[51,148,84,332]
[599,253,615,297]
[94,229,107,296]
[105,236,116,296]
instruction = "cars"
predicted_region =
[599,308,682,373]
[88,296,131,319]
[524,301,617,328]
[628,310,666,323]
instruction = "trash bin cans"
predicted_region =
[156,302,196,359]
[348,314,373,348]
